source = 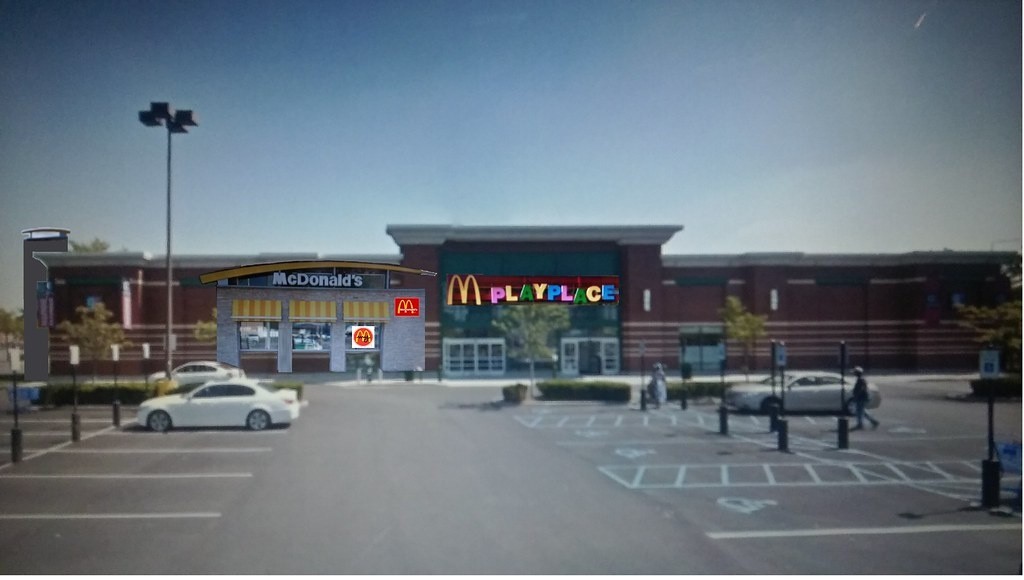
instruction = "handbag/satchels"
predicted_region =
[647,375,657,393]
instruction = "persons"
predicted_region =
[846,365,880,430]
[647,363,668,404]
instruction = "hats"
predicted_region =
[849,366,864,374]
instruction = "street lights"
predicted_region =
[137,100,199,378]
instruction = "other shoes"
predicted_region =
[872,420,879,430]
[854,424,863,429]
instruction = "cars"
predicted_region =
[137,377,301,432]
[148,360,247,386]
[726,369,882,415]
